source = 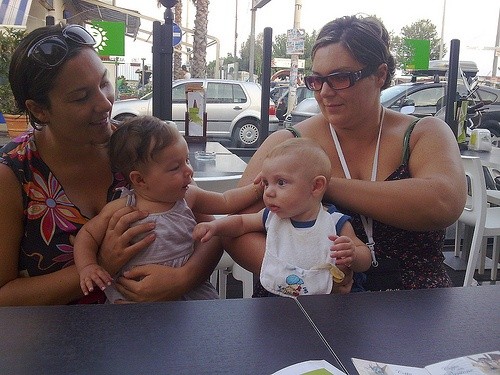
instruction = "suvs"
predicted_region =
[111,78,279,148]
[270,81,315,125]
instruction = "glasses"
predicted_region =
[304,65,371,91]
[16,24,97,112]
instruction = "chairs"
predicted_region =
[457,155,500,287]
[190,175,253,299]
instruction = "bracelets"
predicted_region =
[251,184,260,203]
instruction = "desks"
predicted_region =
[190,141,247,193]
[297,286,499,375]
[0,296,346,375]
[442,145,500,287]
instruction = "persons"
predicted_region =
[178,65,189,79]
[223,14,467,298]
[74,115,266,304]
[136,65,152,89]
[192,137,372,297]
[0,21,224,307]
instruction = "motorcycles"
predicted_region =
[400,66,500,190]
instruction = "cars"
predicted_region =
[283,60,500,148]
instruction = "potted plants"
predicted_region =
[0,24,30,137]
